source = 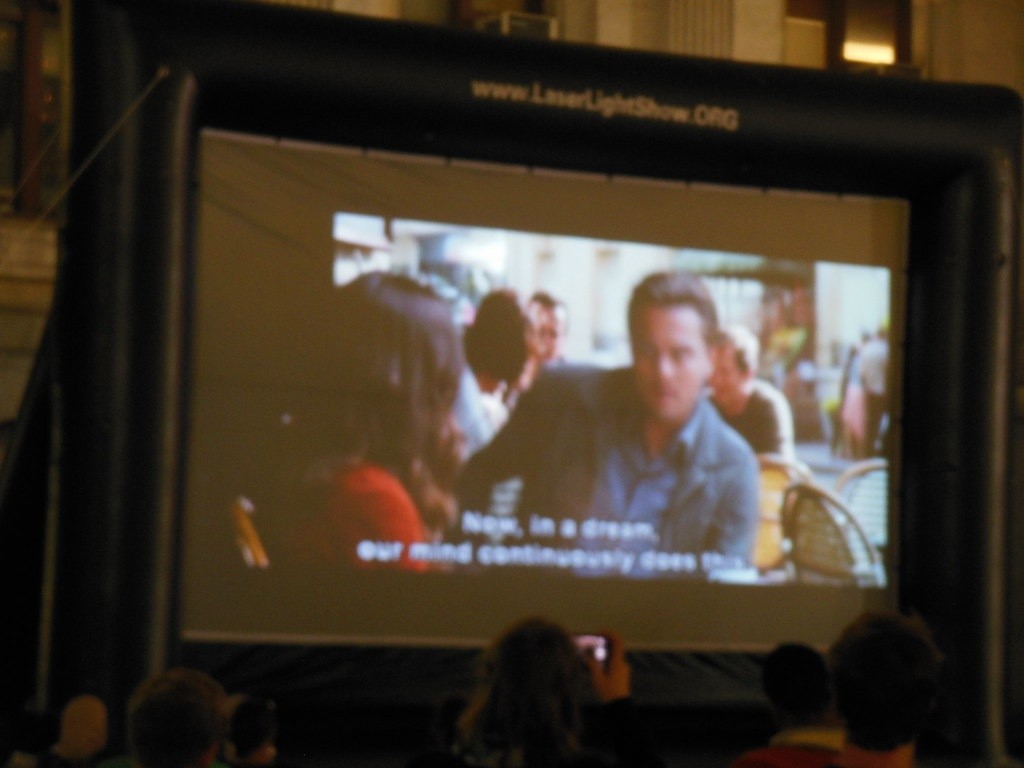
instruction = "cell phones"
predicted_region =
[573,634,610,663]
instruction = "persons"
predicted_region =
[1,618,1024,768]
[321,271,893,585]
